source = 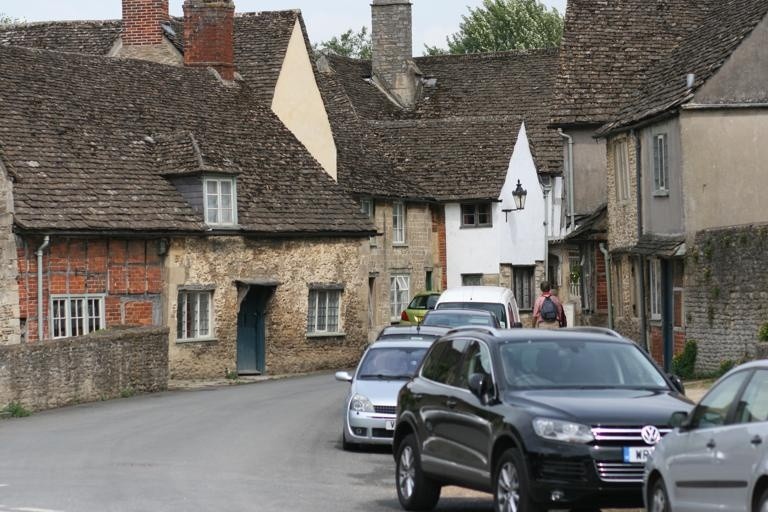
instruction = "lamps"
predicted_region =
[500,178,526,212]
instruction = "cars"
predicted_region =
[642,358,767,511]
[400,290,442,325]
[334,307,499,452]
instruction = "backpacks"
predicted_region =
[541,295,556,322]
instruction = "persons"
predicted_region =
[532,281,563,329]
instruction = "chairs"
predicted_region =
[535,347,562,378]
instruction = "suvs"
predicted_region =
[434,285,525,328]
[391,323,722,512]
